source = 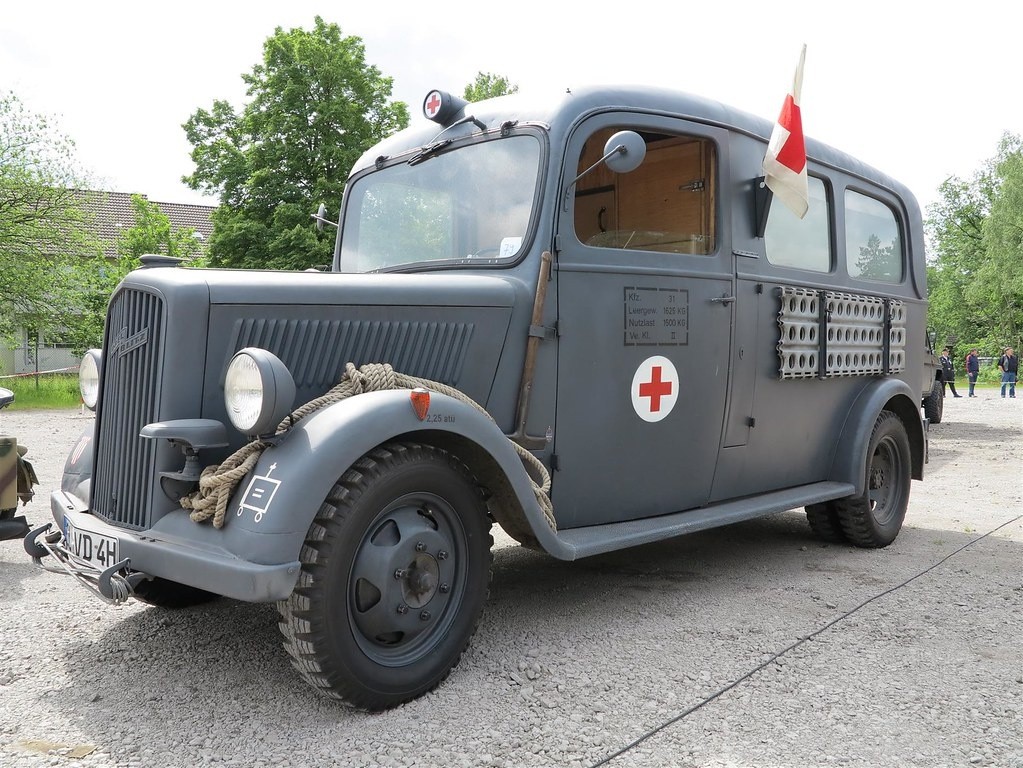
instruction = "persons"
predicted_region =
[966,348,980,398]
[997,346,1018,397]
[938,349,962,397]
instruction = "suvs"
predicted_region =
[921,323,945,424]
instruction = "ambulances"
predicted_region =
[17,81,933,716]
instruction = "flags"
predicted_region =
[762,51,809,218]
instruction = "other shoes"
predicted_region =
[943,396,945,398]
[1010,395,1015,398]
[969,394,976,397]
[954,395,962,397]
[1001,395,1005,398]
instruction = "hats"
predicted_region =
[943,348,950,351]
